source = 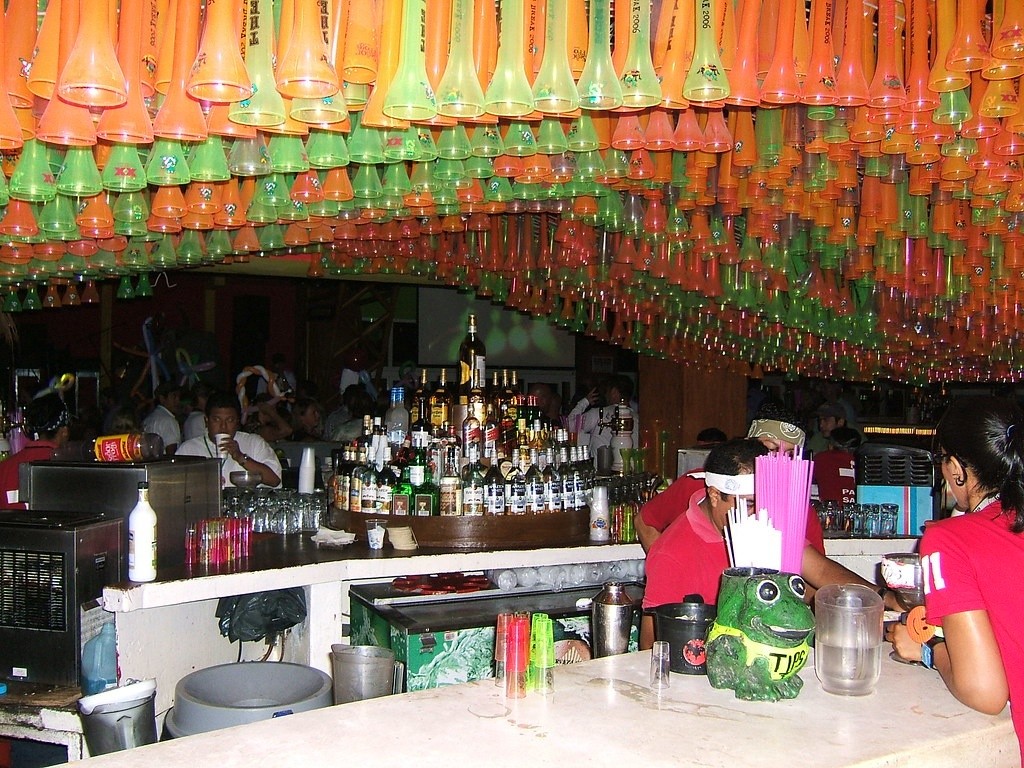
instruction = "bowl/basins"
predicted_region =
[643,601,717,675]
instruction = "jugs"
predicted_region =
[331,643,404,705]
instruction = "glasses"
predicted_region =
[933,453,967,467]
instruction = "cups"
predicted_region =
[811,501,899,536]
[589,486,609,541]
[366,519,387,549]
[215,434,231,459]
[814,583,884,696]
[485,560,646,591]
[650,641,671,689]
[493,610,556,699]
[224,448,324,533]
[620,447,648,478]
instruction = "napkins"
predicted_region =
[310,526,356,544]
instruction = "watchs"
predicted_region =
[920,637,945,669]
[239,453,250,465]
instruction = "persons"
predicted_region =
[812,428,861,511]
[175,393,282,490]
[641,438,907,651]
[142,381,180,456]
[817,403,847,440]
[246,354,385,445]
[102,388,132,434]
[695,429,726,444]
[634,418,824,607]
[570,375,597,415]
[183,386,213,442]
[886,394,1024,768]
[0,394,70,512]
[522,383,562,430]
[566,376,638,473]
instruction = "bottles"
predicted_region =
[51,432,164,462]
[129,481,158,582]
[608,478,669,544]
[0,0,1024,386]
[324,314,593,516]
[81,622,117,695]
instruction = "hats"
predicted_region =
[830,427,861,447]
[22,392,71,435]
[747,400,807,446]
[811,401,847,419]
